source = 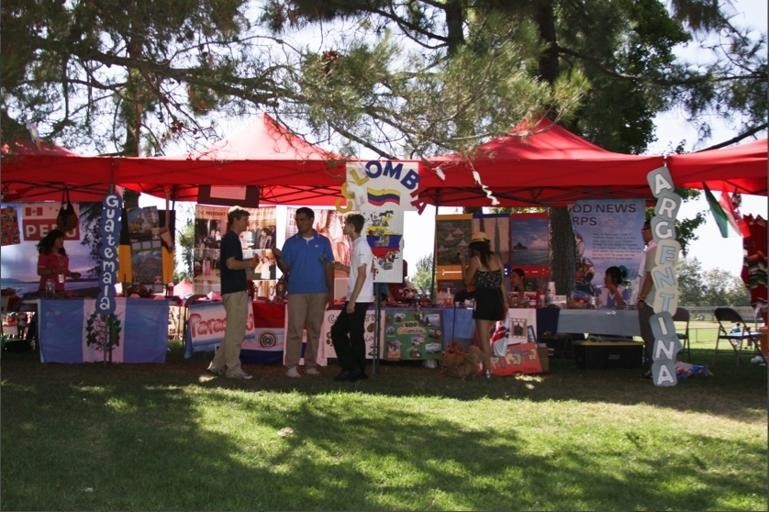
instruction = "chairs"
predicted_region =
[667,306,694,363]
[711,305,766,371]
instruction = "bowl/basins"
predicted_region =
[528,300,536,306]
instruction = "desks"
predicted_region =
[1,295,181,371]
[181,300,479,370]
[552,310,643,354]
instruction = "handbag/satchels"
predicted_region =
[492,325,510,357]
[57,186,78,231]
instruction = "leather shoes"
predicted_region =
[334,369,367,383]
[304,368,321,376]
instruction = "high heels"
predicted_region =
[484,368,493,380]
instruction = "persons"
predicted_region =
[455,281,479,303]
[329,210,374,382]
[730,322,753,352]
[593,266,633,310]
[204,206,260,380]
[511,268,537,292]
[457,233,506,380]
[635,220,655,380]
[198,225,278,281]
[35,228,81,295]
[271,206,335,379]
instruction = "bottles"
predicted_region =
[445,287,457,305]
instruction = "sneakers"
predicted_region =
[207,361,253,381]
[284,367,302,379]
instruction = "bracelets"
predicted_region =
[637,298,644,303]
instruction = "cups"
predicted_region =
[46,280,56,294]
[546,281,556,294]
